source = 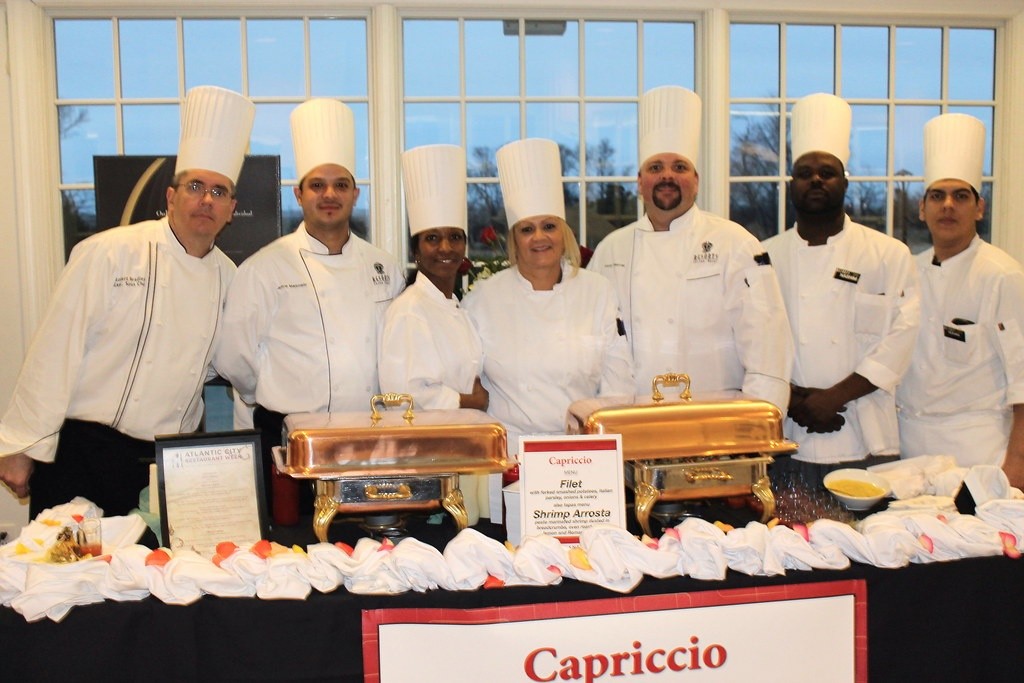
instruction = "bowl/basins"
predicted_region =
[650,500,710,527]
[822,466,891,511]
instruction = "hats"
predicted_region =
[922,113,986,195]
[496,139,565,229]
[402,145,468,235]
[176,87,255,186]
[790,92,851,169]
[289,98,356,186]
[637,85,702,173]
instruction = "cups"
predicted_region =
[77,519,102,560]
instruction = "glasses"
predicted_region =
[177,180,233,203]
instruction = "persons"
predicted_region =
[209,98,408,464]
[462,137,634,458]
[586,85,795,423]
[0,85,256,551]
[377,143,491,411]
[760,92,921,472]
[896,113,1024,490]
